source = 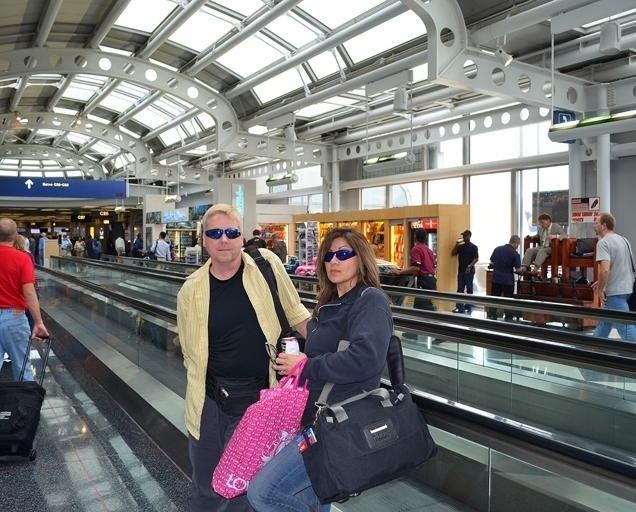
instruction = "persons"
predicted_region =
[451,230,479,314]
[588,213,636,343]
[487,235,522,322]
[247,228,393,512]
[247,230,288,264]
[392,228,438,311]
[515,213,569,278]
[0,218,172,382]
[177,204,313,512]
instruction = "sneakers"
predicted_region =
[452,307,471,313]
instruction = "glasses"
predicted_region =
[203,228,242,239]
[324,248,359,261]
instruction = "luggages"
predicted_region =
[0,334,54,461]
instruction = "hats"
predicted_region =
[460,230,472,236]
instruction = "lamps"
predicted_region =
[392,78,408,113]
[263,107,299,188]
[540,14,636,144]
[597,16,628,56]
[359,78,417,174]
[492,12,517,67]
[283,120,298,144]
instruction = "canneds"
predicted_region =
[280,337,300,356]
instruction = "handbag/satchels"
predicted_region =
[210,358,310,499]
[299,384,439,506]
[149,251,154,260]
[626,277,636,311]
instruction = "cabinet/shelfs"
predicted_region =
[513,281,602,330]
[291,204,471,313]
[484,265,507,314]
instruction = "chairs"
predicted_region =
[523,236,562,281]
[563,238,600,282]
